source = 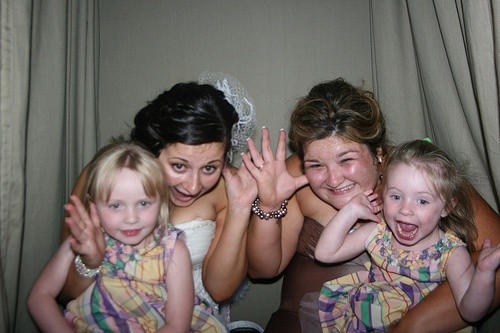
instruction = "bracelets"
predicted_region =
[251,198,289,220]
[74,254,103,277]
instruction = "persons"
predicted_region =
[27,140,229,333]
[240,78,500,333]
[63,71,263,312]
[299,138,500,333]
